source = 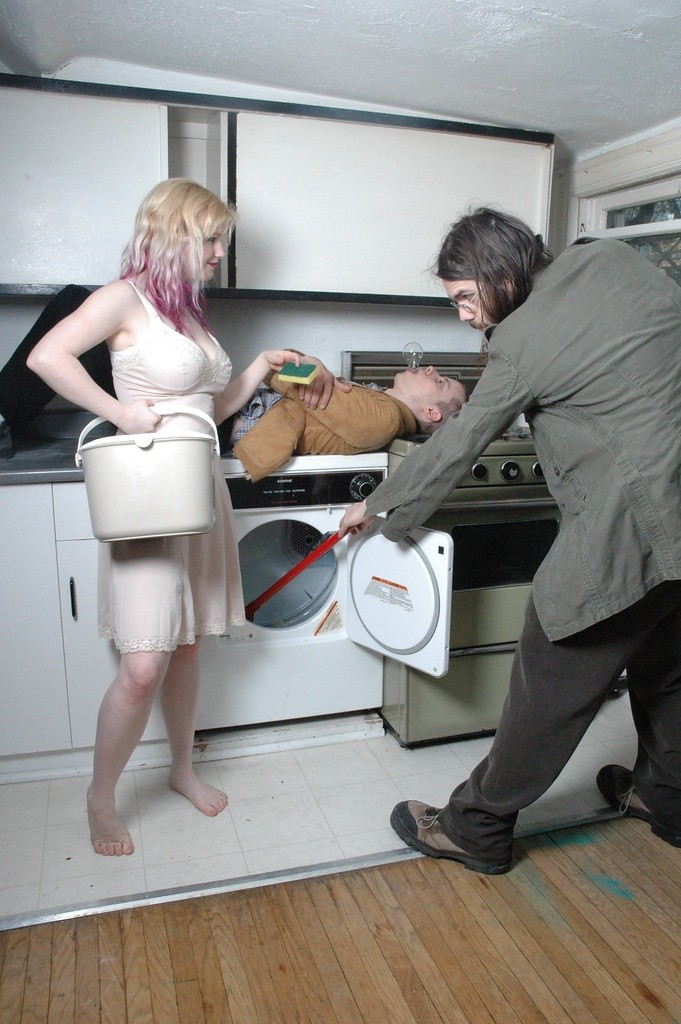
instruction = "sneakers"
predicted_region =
[596,764,681,848]
[390,799,511,875]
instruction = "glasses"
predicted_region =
[450,292,479,312]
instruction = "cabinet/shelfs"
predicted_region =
[2,71,229,299]
[1,484,171,758]
[231,97,555,308]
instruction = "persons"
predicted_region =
[0,284,469,483]
[23,177,305,855]
[334,205,681,876]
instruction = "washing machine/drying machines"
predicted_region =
[181,450,455,734]
